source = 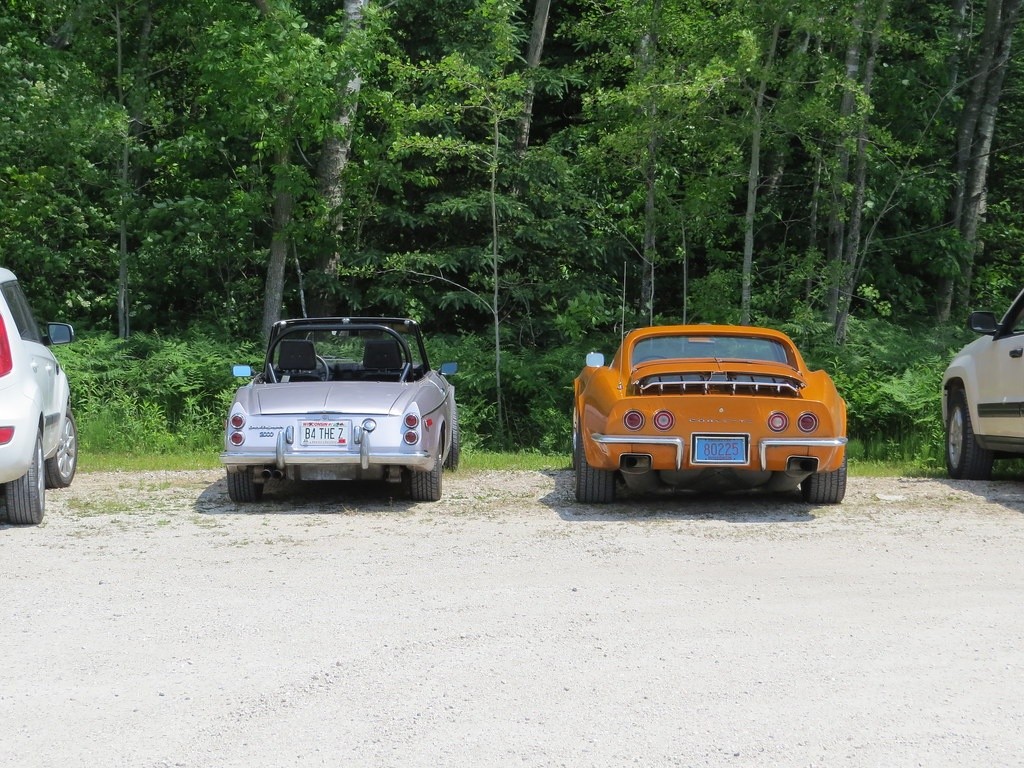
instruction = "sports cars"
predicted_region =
[220,316,462,504]
[572,322,848,507]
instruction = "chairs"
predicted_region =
[277,338,323,382]
[362,339,402,382]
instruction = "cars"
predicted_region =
[0,267,81,529]
[938,287,1023,478]
